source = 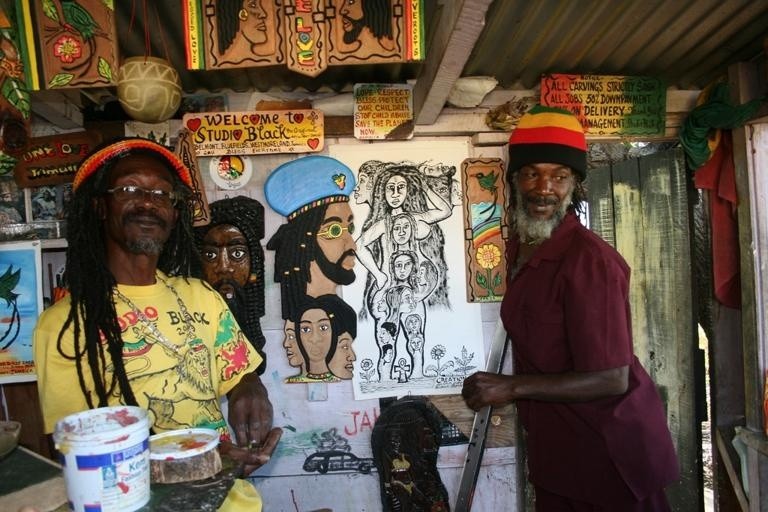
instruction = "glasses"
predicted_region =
[105,184,180,209]
[316,223,354,238]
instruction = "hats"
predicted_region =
[506,106,586,182]
[72,136,196,195]
[263,155,355,221]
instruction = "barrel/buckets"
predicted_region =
[52,405,154,512]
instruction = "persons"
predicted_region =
[462,104,681,512]
[32,135,284,481]
[280,315,307,384]
[352,161,463,383]
[335,1,396,54]
[314,293,356,380]
[209,1,269,68]
[287,301,341,383]
[192,194,267,352]
[263,155,358,320]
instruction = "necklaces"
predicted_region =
[112,276,212,381]
